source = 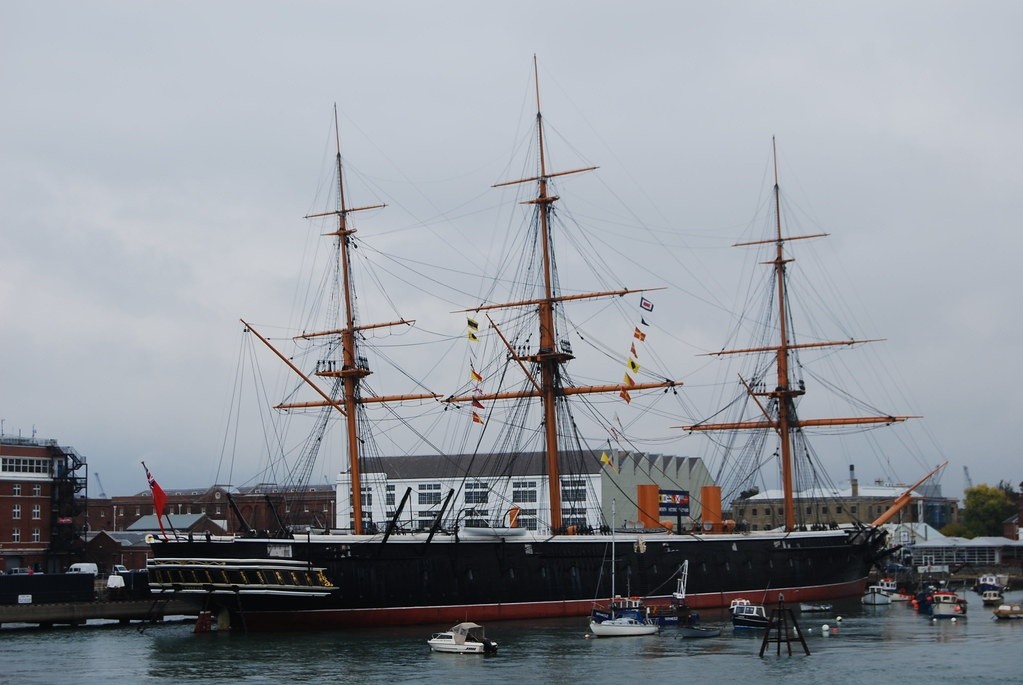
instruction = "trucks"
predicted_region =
[100,565,127,578]
[2,568,44,577]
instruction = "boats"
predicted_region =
[729,600,751,614]
[800,604,832,611]
[590,498,660,636]
[861,591,891,604]
[975,574,1003,594]
[426,622,498,653]
[592,560,698,627]
[983,591,1004,605]
[733,605,783,630]
[930,591,967,617]
[993,604,1023,618]
[679,625,720,638]
[869,577,915,600]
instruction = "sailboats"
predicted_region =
[142,53,949,632]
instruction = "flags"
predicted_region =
[640,297,654,312]
[471,370,482,382]
[146,466,170,541]
[619,373,635,404]
[634,327,646,342]
[467,317,478,331]
[627,357,640,374]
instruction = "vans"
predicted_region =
[65,563,98,577]
